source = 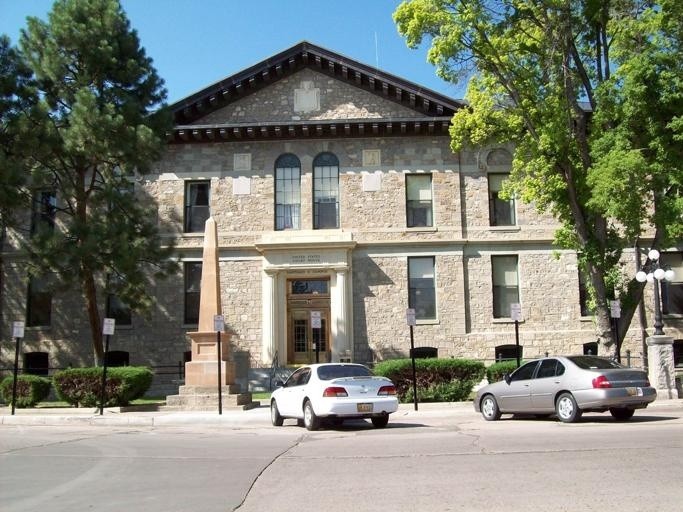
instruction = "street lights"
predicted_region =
[635,250,675,336]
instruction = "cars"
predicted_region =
[271,362,399,432]
[474,355,656,422]
